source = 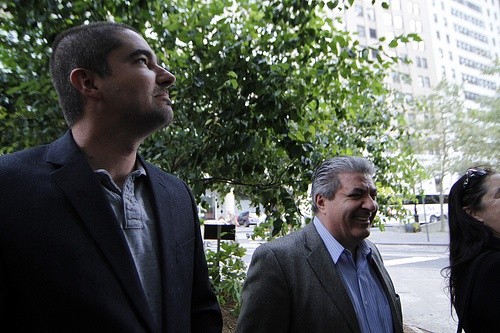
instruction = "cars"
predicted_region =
[237,210,262,227]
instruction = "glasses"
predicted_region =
[463,167,490,192]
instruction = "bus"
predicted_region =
[371,191,449,228]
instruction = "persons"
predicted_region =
[440,165,500,333]
[235,154,404,333]
[0,21,224,333]
